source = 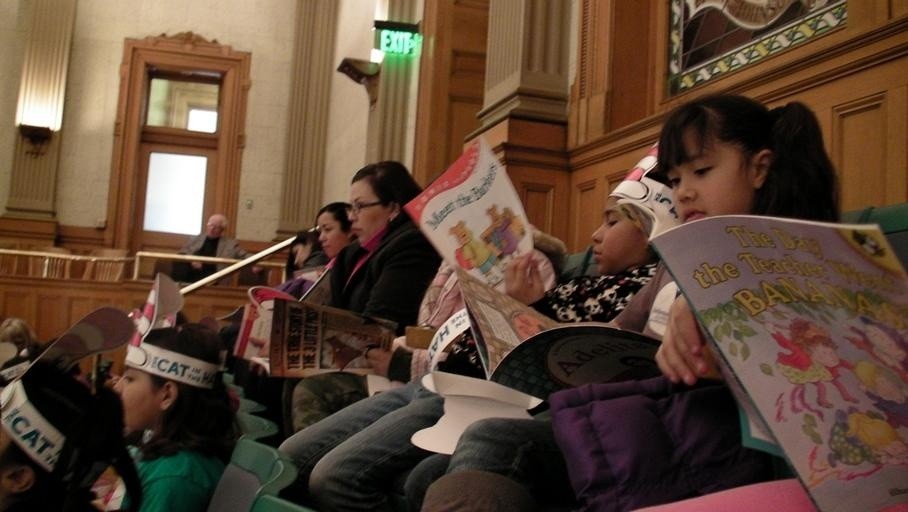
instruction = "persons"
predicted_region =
[0,92,906,511]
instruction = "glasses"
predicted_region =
[346,202,381,215]
